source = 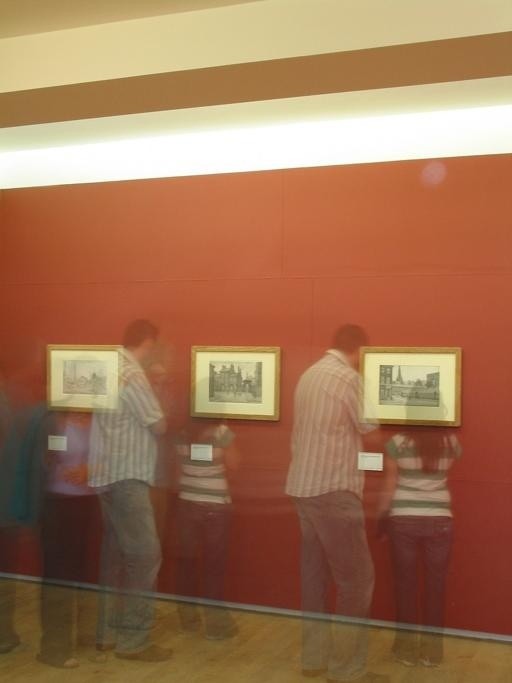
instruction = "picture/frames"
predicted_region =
[47,342,126,416]
[357,346,462,429]
[189,344,282,421]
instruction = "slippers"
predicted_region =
[37,653,79,667]
[77,648,105,663]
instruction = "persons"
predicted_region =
[281,319,378,680]
[2,381,40,662]
[31,358,105,672]
[370,375,461,667]
[85,315,170,667]
[169,380,243,640]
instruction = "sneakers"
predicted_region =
[422,657,441,668]
[395,654,420,667]
[96,643,117,650]
[169,616,199,633]
[1,636,22,651]
[303,670,332,674]
[116,645,172,661]
[108,601,166,630]
[330,670,389,682]
[204,628,238,640]
